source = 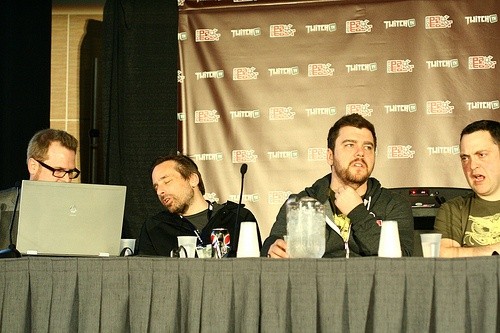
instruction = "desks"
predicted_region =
[0,256,500,333]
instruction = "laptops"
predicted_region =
[15,180,127,257]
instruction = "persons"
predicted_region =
[137,155,262,258]
[434,120,500,258]
[260,113,414,258]
[0,128,81,251]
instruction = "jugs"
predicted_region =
[286,196,327,259]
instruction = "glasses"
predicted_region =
[34,158,81,179]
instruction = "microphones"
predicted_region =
[0,180,21,258]
[221,163,248,258]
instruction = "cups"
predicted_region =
[177,235,198,258]
[236,222,261,259]
[419,234,442,258]
[196,243,213,259]
[377,221,402,258]
[120,239,136,256]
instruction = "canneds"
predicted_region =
[211,228,231,258]
